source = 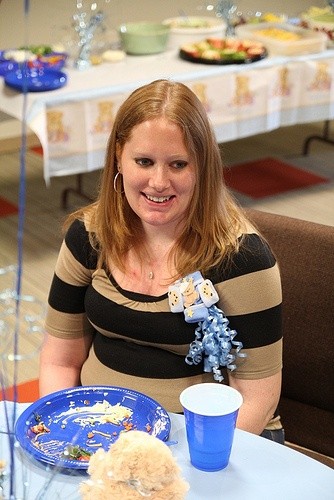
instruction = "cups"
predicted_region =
[179,380,244,472]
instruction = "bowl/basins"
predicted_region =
[118,21,169,54]
[164,16,227,49]
[0,47,68,76]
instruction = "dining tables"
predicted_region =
[0,401,334,500]
[0,16,334,208]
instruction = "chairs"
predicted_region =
[244,208,334,458]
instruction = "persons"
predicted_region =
[38,79,283,436]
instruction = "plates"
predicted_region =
[179,37,267,64]
[6,68,67,90]
[15,385,172,472]
